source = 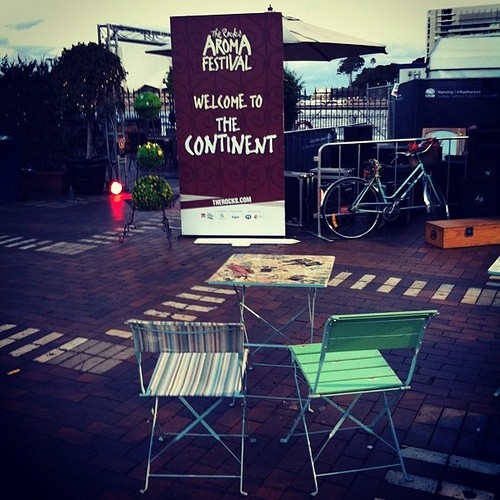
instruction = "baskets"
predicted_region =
[410,147,442,170]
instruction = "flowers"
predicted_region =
[134,91,162,107]
[123,175,181,200]
[137,142,164,158]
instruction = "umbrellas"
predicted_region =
[145,3,389,61]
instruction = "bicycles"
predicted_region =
[320,137,452,239]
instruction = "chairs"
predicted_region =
[280,310,440,496]
[127,320,256,496]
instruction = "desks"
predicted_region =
[208,253,336,413]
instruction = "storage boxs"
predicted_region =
[373,181,413,210]
[425,216,500,249]
[310,167,355,201]
[284,171,315,228]
